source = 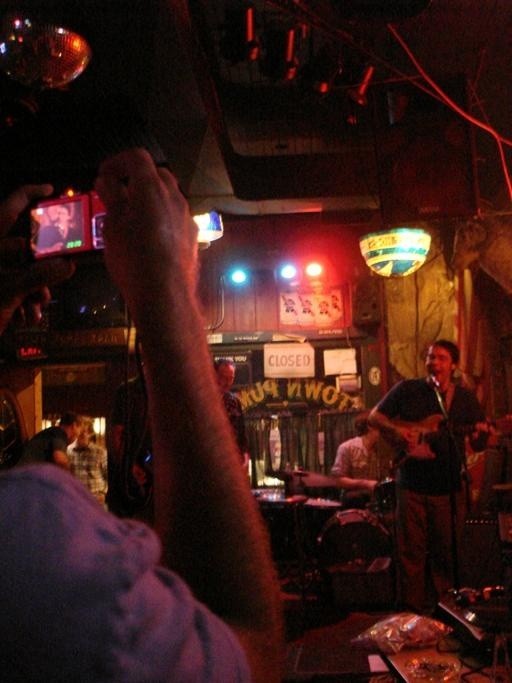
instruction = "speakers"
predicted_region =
[351,278,381,326]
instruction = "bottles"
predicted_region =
[283,463,294,496]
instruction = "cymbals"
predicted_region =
[265,470,334,488]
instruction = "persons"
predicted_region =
[369,338,466,610]
[332,414,388,507]
[2,151,282,682]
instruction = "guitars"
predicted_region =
[124,415,154,508]
[378,411,512,468]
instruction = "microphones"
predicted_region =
[426,369,441,387]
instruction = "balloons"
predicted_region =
[0,10,91,91]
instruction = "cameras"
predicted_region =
[18,191,110,258]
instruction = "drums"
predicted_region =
[260,494,393,583]
[374,479,397,514]
